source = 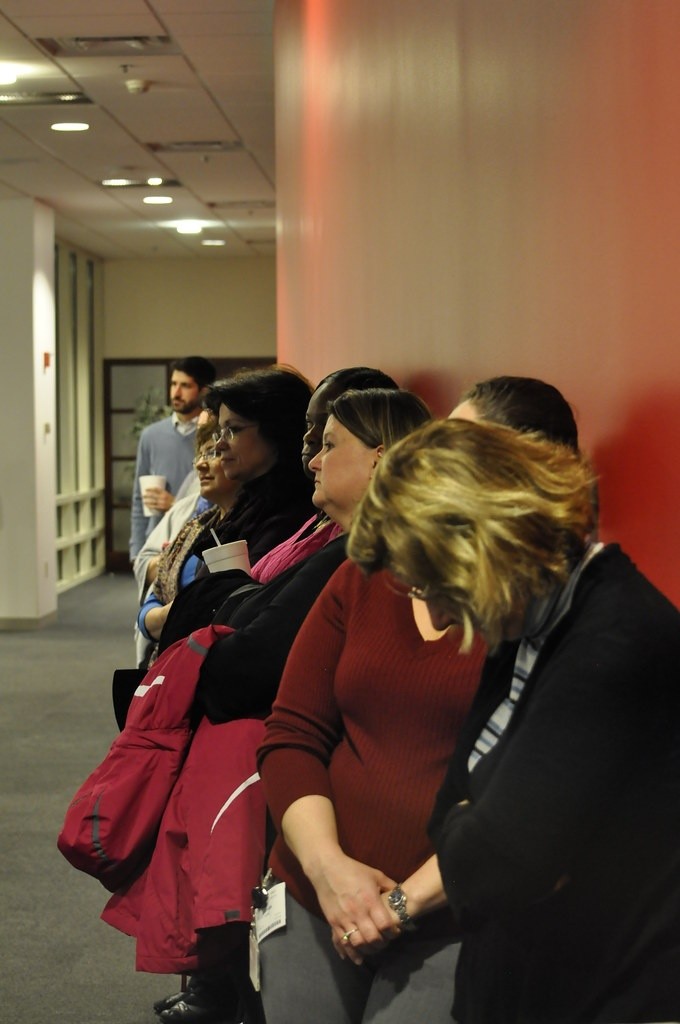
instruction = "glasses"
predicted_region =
[191,449,221,469]
[213,422,260,446]
[409,583,431,600]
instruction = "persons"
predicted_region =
[257,375,578,1024]
[345,417,680,1024]
[128,357,218,562]
[131,363,435,1024]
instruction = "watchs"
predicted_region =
[389,884,411,924]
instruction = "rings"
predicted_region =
[341,928,358,940]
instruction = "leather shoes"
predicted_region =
[159,993,214,1024]
[153,987,195,1013]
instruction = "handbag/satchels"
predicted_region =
[112,670,157,734]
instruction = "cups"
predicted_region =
[203,540,251,582]
[139,474,166,518]
